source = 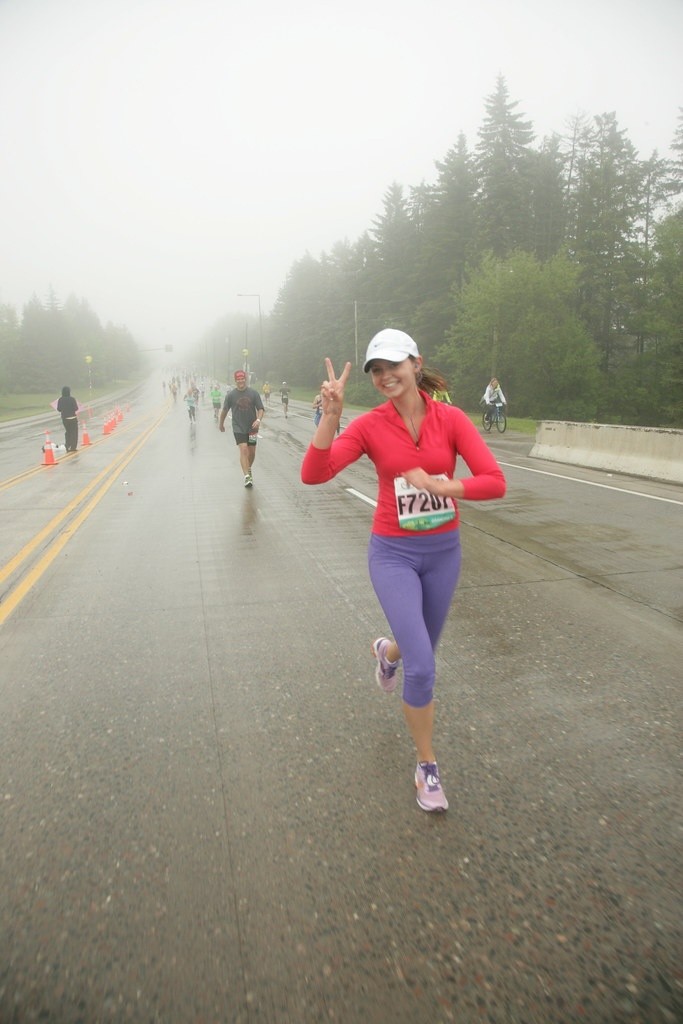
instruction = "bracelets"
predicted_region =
[257,419,261,422]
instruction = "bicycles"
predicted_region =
[482,401,508,434]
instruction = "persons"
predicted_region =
[278,382,290,418]
[484,378,506,422]
[301,328,508,814]
[162,367,232,423]
[263,381,271,405]
[219,370,265,487]
[313,395,340,436]
[433,379,452,404]
[57,386,78,451]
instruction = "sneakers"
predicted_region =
[416,758,449,814]
[244,476,253,487]
[371,637,399,693]
[249,469,253,480]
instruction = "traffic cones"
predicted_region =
[87,405,94,420]
[101,403,130,436]
[80,418,93,445]
[40,429,60,465]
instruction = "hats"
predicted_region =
[234,370,246,381]
[363,329,419,373]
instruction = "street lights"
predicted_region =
[235,292,265,366]
